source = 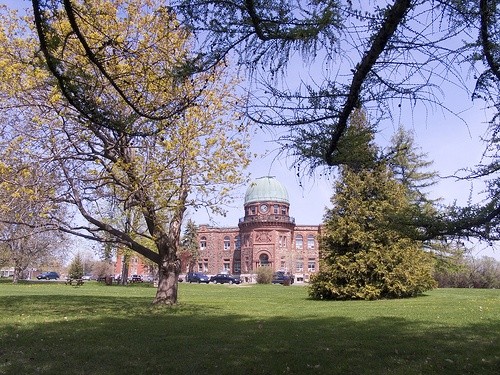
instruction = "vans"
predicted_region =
[270,271,294,285]
[184,272,210,284]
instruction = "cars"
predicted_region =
[71,272,155,283]
[211,273,243,284]
[36,271,59,280]
